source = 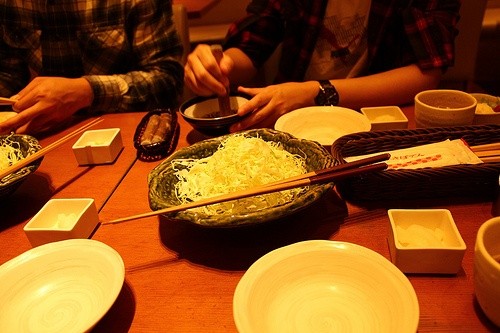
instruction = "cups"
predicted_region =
[415,89,478,128]
[468,93,500,126]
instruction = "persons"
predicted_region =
[183,0,459,134]
[0,0,183,134]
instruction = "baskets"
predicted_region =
[331,123,500,200]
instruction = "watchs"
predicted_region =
[316,79,339,106]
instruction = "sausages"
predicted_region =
[141,113,172,145]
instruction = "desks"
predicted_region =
[0,105,500,333]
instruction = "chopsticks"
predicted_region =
[98,152,392,225]
[0,116,104,180]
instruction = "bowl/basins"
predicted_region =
[72,126,124,165]
[360,106,409,131]
[0,238,126,333]
[0,133,46,189]
[387,208,468,275]
[274,106,371,154]
[474,216,500,327]
[22,198,100,248]
[179,90,257,138]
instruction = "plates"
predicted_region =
[231,240,421,333]
